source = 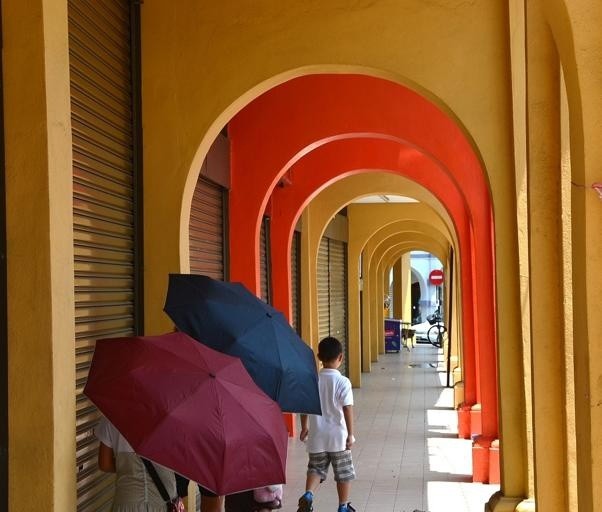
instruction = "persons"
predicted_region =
[94,416,184,512]
[297,338,356,512]
[175,473,223,512]
[225,484,283,512]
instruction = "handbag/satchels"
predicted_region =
[164,494,186,512]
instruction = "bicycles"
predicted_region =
[428,300,446,351]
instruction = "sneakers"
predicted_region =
[337,501,358,512]
[296,491,315,512]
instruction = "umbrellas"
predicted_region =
[83,330,290,497]
[163,274,322,415]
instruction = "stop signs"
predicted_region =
[429,269,444,286]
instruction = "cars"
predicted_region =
[410,321,444,343]
